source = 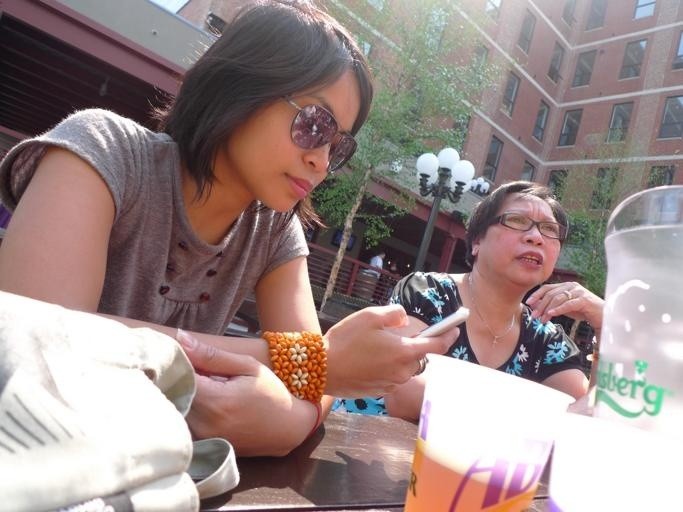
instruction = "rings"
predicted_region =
[564,290,572,301]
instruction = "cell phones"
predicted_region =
[409,306,470,338]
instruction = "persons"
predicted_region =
[382,176,605,426]
[0,2,471,460]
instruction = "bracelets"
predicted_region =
[261,328,327,404]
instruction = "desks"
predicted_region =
[186,415,561,512]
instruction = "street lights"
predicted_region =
[411,144,492,273]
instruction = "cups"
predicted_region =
[403,352,577,512]
[582,184,683,511]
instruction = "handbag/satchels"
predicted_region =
[0,289,241,511]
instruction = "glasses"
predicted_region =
[282,96,358,174]
[490,211,568,240]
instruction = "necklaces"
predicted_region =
[466,273,515,348]
